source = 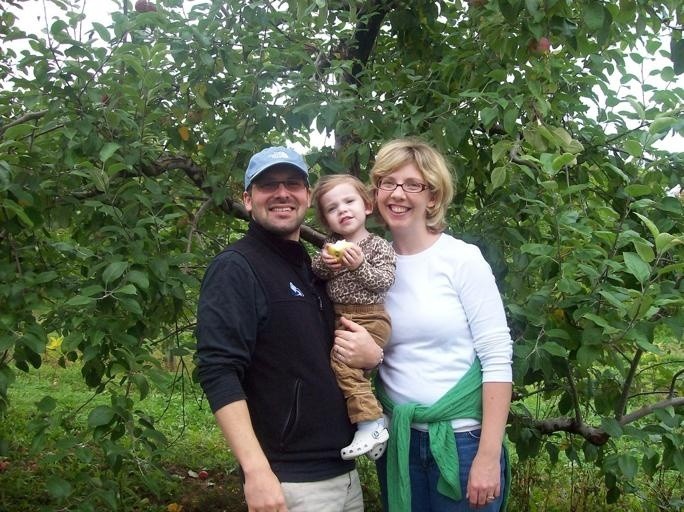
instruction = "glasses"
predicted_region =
[255,177,305,194]
[378,179,430,193]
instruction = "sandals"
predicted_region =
[366,413,388,459]
[340,424,389,460]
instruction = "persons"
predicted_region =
[311,173,396,461]
[195,146,382,511]
[366,138,513,511]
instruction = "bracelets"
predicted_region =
[364,345,384,370]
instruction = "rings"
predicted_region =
[486,496,495,503]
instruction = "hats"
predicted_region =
[245,146,310,191]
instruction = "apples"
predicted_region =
[135,0,155,12]
[188,106,202,123]
[199,471,208,480]
[532,36,549,54]
[100,93,109,104]
[328,239,353,266]
